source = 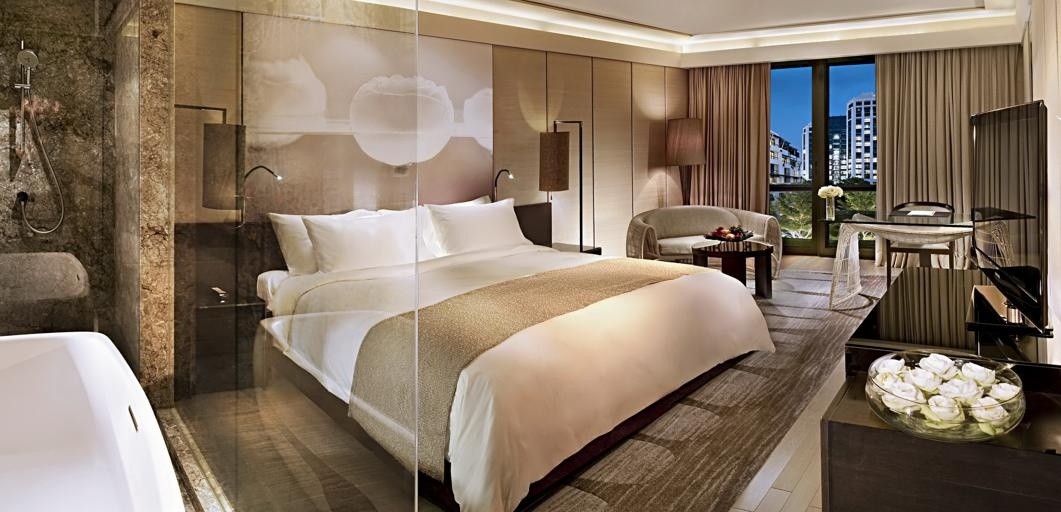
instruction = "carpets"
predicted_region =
[174,265,903,509]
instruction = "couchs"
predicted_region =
[626,206,783,281]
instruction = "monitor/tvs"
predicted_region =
[964,98,1053,339]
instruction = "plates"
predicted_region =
[703,233,754,243]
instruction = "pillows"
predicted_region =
[265,195,537,276]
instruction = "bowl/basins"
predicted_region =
[865,349,1027,446]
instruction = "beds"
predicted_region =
[256,204,761,512]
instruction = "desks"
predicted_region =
[821,211,974,317]
[975,207,1037,268]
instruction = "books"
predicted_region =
[906,210,936,217]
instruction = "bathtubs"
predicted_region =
[0,331,184,512]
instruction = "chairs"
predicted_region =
[888,201,956,292]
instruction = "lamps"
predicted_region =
[174,102,247,210]
[541,119,582,253]
[668,116,709,206]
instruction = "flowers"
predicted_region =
[873,354,1024,437]
[818,185,844,216]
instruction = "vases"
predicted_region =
[825,195,836,221]
